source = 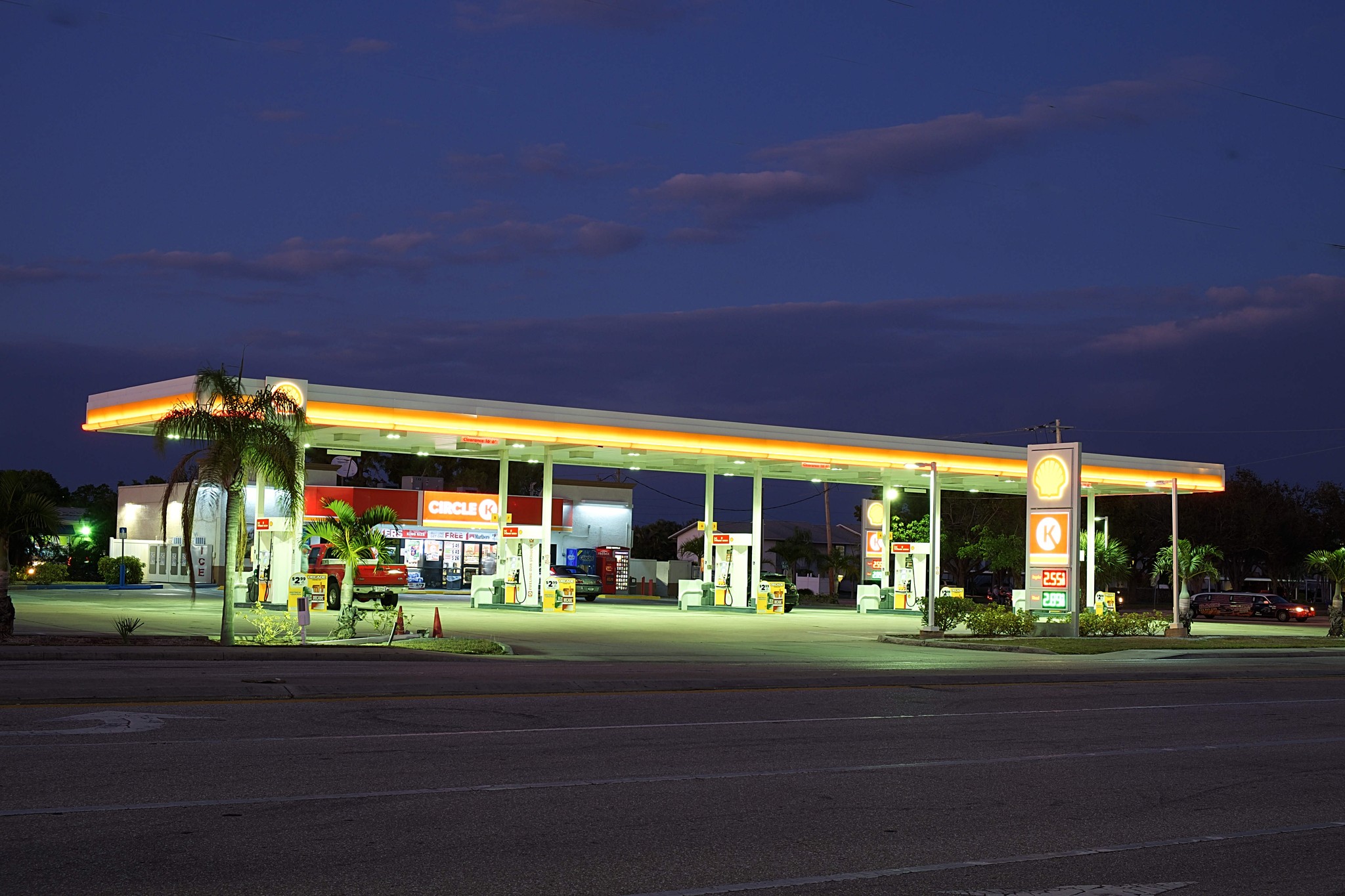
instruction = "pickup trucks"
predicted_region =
[307,544,409,610]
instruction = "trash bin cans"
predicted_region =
[245,577,258,603]
[446,567,461,590]
[492,578,505,604]
[879,586,894,610]
[629,577,637,595]
[701,582,715,606]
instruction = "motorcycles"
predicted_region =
[987,592,1012,606]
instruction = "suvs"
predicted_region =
[747,570,799,612]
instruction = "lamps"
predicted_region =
[770,465,792,472]
[1081,482,1098,488]
[964,485,984,492]
[940,477,963,484]
[718,468,740,476]
[569,451,594,459]
[380,429,406,438]
[456,442,482,452]
[1148,487,1169,494]
[914,469,939,476]
[727,455,753,464]
[410,446,435,456]
[999,475,1022,482]
[505,439,532,447]
[623,462,647,470]
[1094,490,1111,495]
[304,439,318,447]
[333,432,360,443]
[673,458,698,468]
[805,474,827,482]
[830,464,848,470]
[858,472,881,479]
[890,480,909,486]
[621,448,647,456]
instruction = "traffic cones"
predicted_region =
[433,607,444,638]
[394,606,404,635]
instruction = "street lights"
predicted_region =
[903,462,944,639]
[1146,478,1189,639]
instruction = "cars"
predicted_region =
[1173,593,1315,623]
[538,565,602,602]
[1094,585,1123,612]
